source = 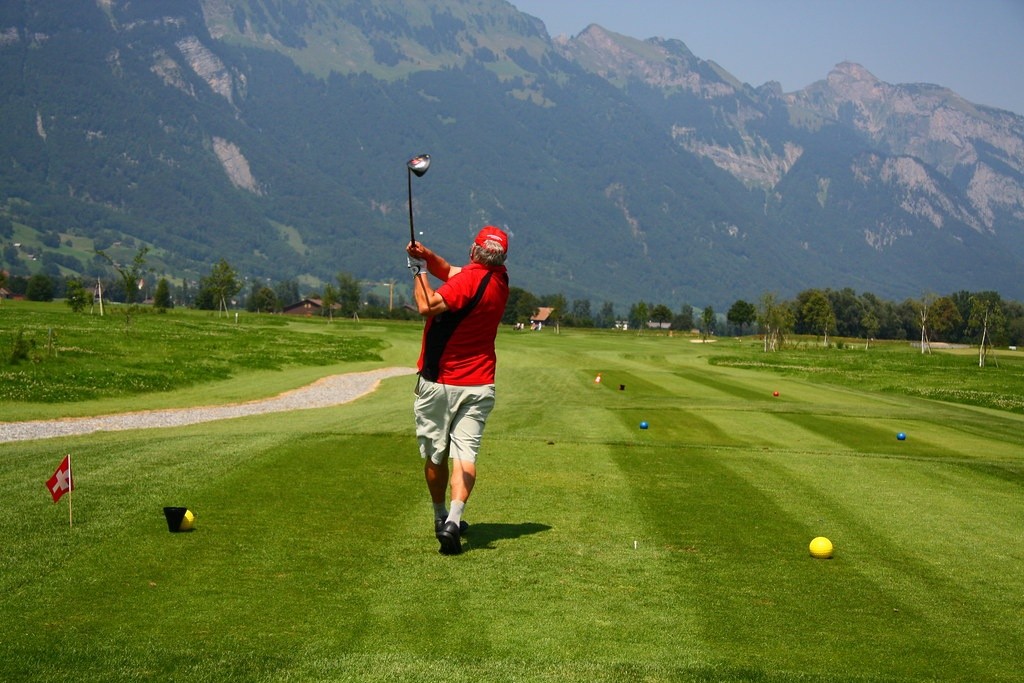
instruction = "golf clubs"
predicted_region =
[407,154,432,247]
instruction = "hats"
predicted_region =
[475,225,508,255]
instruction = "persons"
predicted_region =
[405,227,510,556]
[516,321,538,331]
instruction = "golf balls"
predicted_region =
[773,392,779,396]
[640,421,649,429]
[809,537,833,559]
[179,509,195,531]
[896,432,906,441]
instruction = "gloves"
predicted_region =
[407,253,427,279]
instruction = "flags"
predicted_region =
[46,455,74,502]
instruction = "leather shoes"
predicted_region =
[436,521,462,554]
[435,518,468,537]
